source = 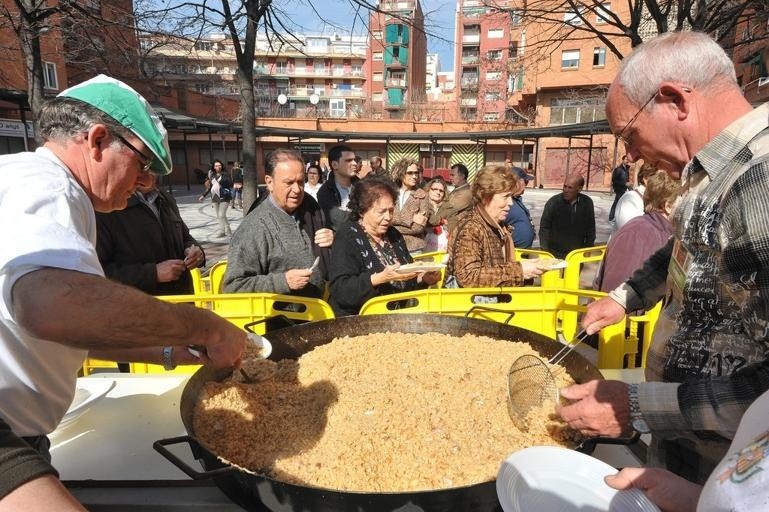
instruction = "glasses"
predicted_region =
[431,187,445,193]
[406,170,418,175]
[615,83,693,145]
[114,131,153,172]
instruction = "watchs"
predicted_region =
[627,384,652,436]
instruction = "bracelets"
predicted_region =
[160,344,178,371]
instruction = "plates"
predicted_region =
[496,445,662,511]
[520,259,568,270]
[393,262,447,274]
[187,332,273,363]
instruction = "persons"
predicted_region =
[196,144,688,369]
[95,169,207,298]
[552,27,768,485]
[602,391,768,512]
[0,70,249,512]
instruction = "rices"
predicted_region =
[191,331,587,493]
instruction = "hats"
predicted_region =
[510,166,535,180]
[54,73,173,177]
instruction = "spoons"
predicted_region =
[308,256,321,273]
[183,243,195,264]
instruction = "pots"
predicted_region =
[152,306,642,512]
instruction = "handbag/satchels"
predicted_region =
[221,187,233,199]
